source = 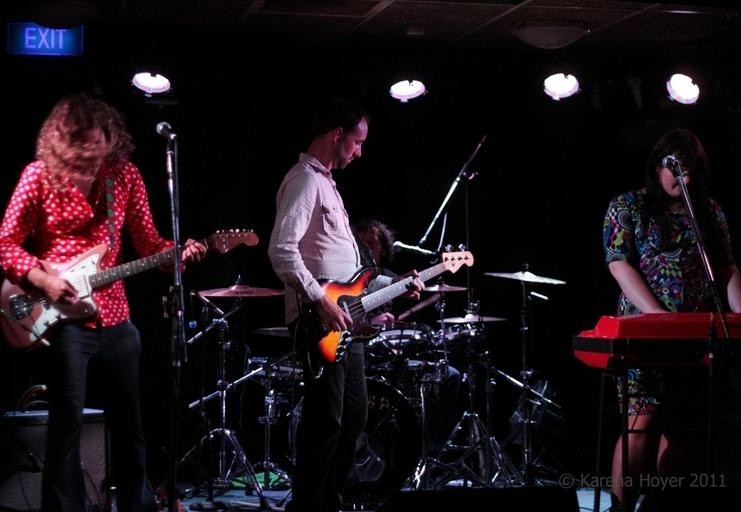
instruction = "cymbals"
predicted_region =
[423,284,468,292]
[190,285,289,296]
[484,271,567,285]
[437,314,507,323]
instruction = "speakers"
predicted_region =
[648,480,741,512]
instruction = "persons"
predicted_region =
[603,129,740,509]
[355,220,394,260]
[269,105,425,510]
[0,99,207,511]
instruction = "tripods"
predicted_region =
[156,286,591,512]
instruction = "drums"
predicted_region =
[247,322,486,492]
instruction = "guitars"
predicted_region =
[0,229,259,348]
[302,244,474,365]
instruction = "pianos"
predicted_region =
[575,312,741,368]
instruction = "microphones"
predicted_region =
[660,154,682,183]
[155,121,177,141]
